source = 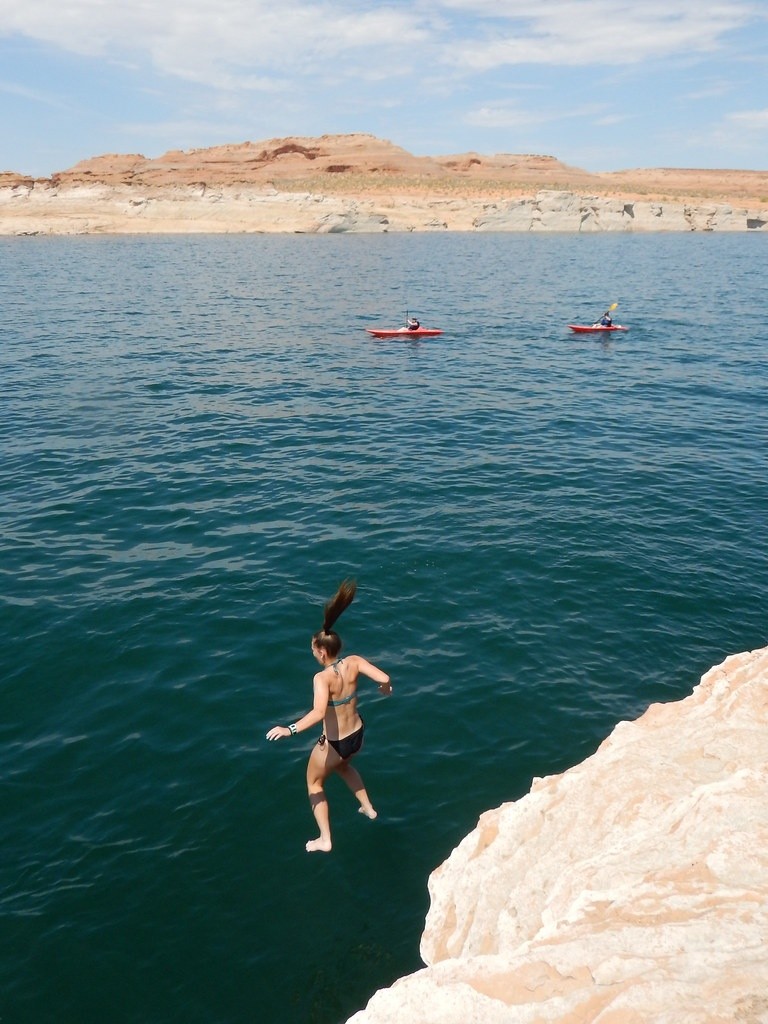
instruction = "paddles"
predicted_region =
[405,309,409,328]
[592,303,618,323]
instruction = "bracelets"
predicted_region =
[288,724,297,735]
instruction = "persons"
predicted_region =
[398,317,419,331]
[594,313,613,327]
[266,579,392,852]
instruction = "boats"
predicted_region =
[365,324,447,338]
[567,323,631,333]
